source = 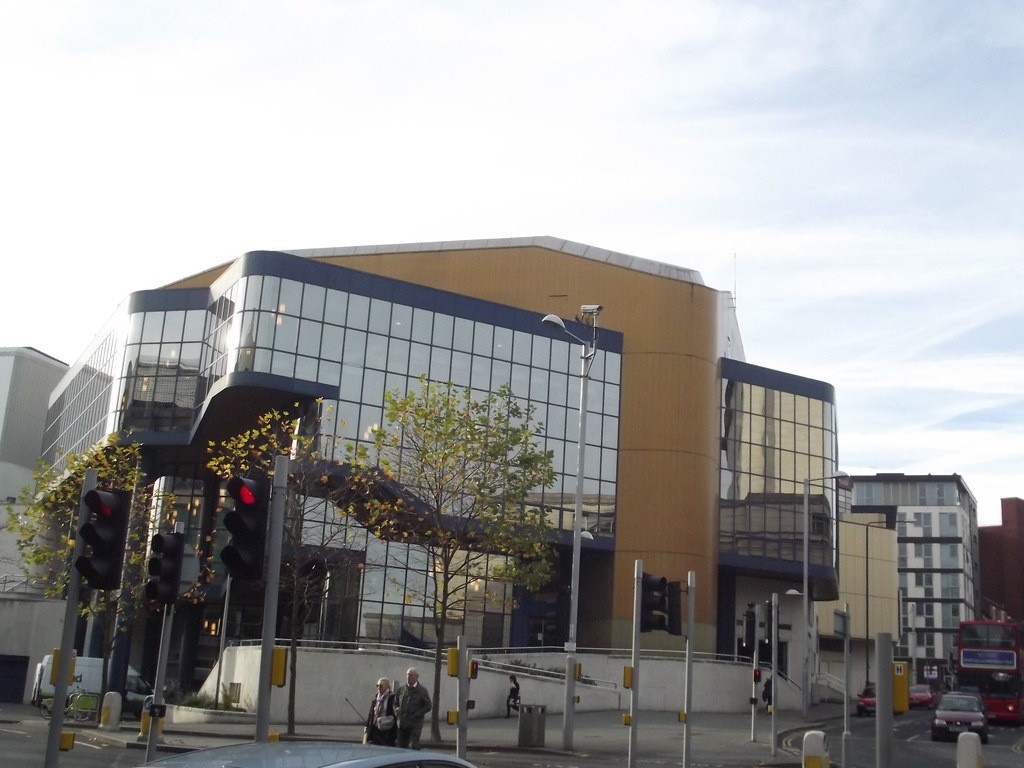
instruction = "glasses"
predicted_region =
[376,685,382,687]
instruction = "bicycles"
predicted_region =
[39,686,91,721]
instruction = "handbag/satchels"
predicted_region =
[377,715,395,730]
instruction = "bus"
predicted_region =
[957,621,1024,724]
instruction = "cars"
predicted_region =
[931,690,989,744]
[909,682,939,711]
[856,681,876,717]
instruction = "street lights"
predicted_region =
[541,314,591,749]
[865,519,919,689]
[801,470,849,713]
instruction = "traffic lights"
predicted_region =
[219,475,272,580]
[469,659,478,679]
[623,665,631,688]
[753,668,762,682]
[678,711,685,722]
[75,485,132,591]
[576,662,582,680]
[142,530,185,603]
[273,646,288,687]
[447,709,457,723]
[643,574,682,635]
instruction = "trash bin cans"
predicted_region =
[518,704,546,747]
[137,695,167,742]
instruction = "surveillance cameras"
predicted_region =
[580,305,603,314]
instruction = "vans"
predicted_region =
[31,655,167,718]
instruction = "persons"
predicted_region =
[764,676,773,715]
[505,675,520,718]
[366,679,399,747]
[393,666,431,751]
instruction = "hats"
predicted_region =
[509,675,516,679]
